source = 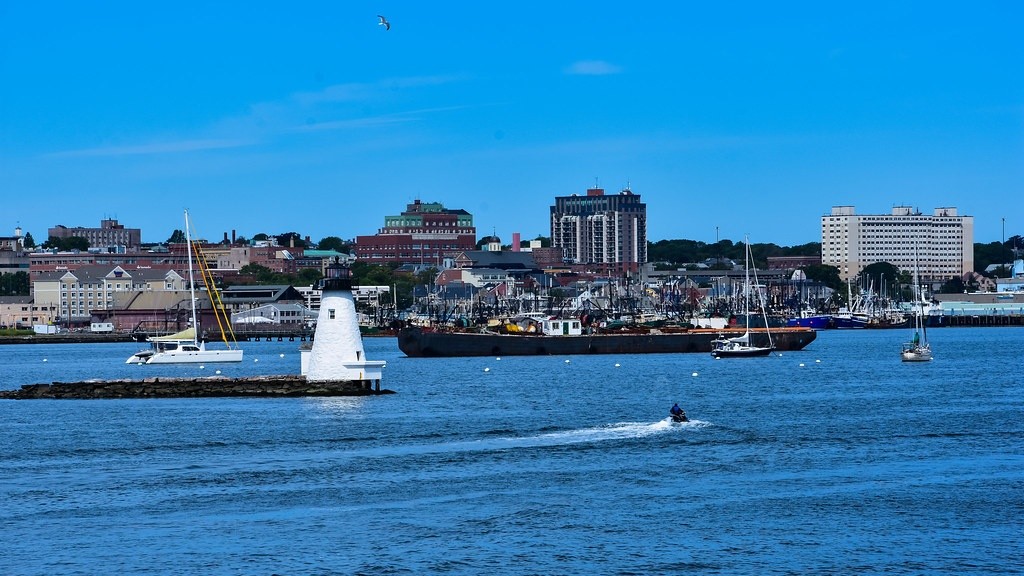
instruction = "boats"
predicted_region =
[669,410,688,424]
[396,326,819,359]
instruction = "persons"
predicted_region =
[791,289,800,305]
[776,292,781,305]
[669,403,684,420]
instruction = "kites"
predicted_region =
[376,15,391,31]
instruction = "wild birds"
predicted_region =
[378,15,390,31]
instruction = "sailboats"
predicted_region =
[358,268,930,334]
[710,232,778,358]
[125,206,243,364]
[901,232,933,362]
